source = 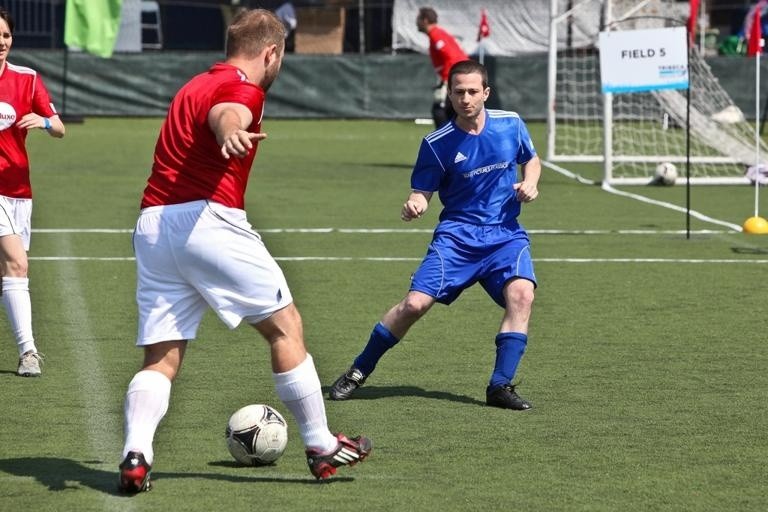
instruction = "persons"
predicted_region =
[329,61,541,411]
[0,12,65,377]
[120,8,371,494]
[417,7,470,130]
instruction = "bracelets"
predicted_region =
[44,117,52,129]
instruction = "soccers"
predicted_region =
[225,403,288,466]
[743,216,768,235]
[654,161,677,187]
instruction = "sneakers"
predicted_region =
[486,384,532,410]
[305,433,372,480]
[330,369,363,401]
[18,353,42,376]
[118,450,152,495]
